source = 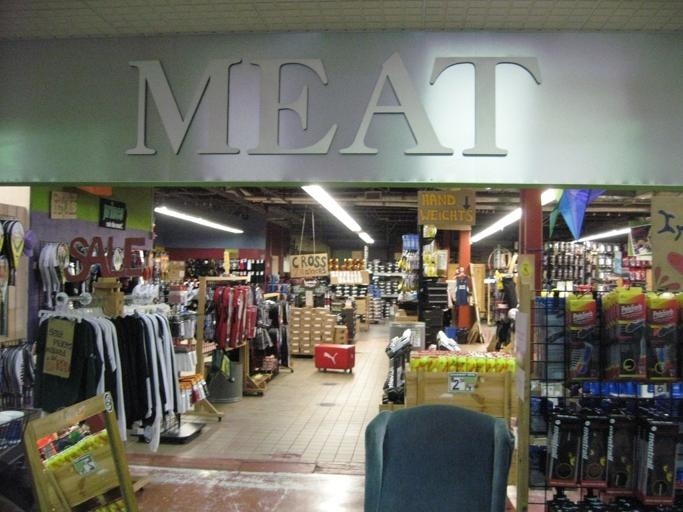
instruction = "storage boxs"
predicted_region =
[287,305,336,356]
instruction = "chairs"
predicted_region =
[363,406,513,512]
[20,391,138,512]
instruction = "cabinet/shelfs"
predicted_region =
[327,247,404,331]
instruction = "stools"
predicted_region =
[314,342,355,375]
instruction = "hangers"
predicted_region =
[36,294,168,327]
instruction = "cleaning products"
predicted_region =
[566,285,682,345]
